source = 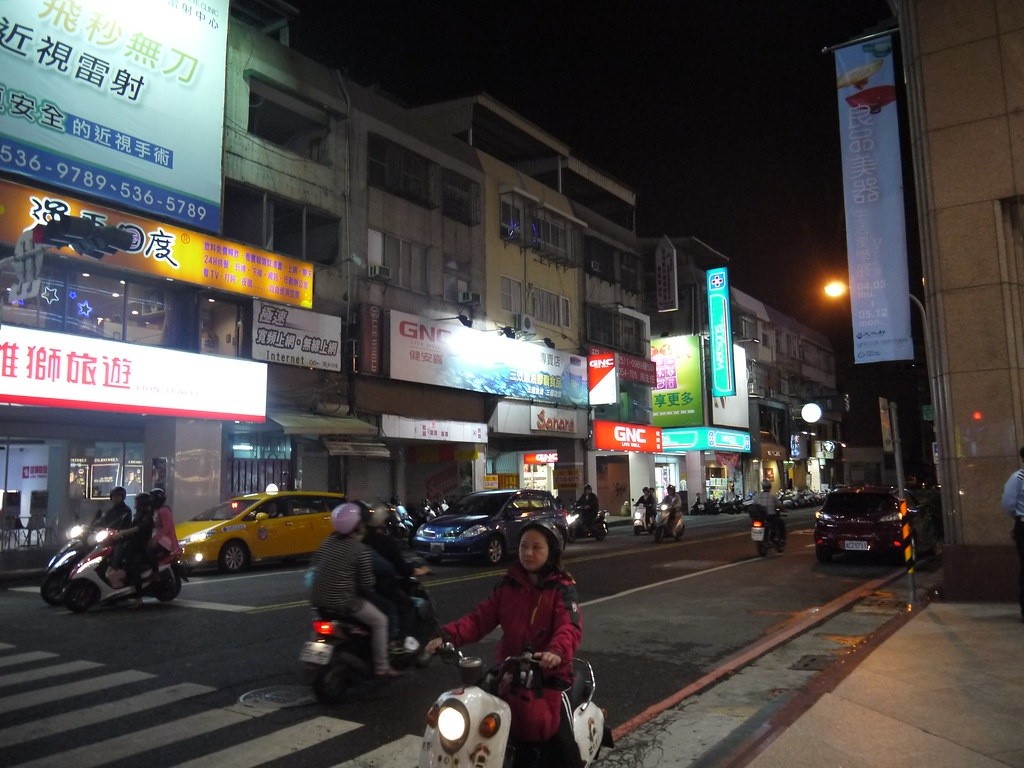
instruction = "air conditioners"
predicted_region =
[591,260,600,272]
[368,265,391,280]
[458,291,481,304]
[515,313,536,333]
[748,383,760,394]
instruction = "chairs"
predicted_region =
[0,514,46,551]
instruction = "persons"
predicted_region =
[264,500,284,519]
[311,500,432,674]
[424,520,584,768]
[571,485,599,537]
[92,487,179,610]
[632,484,683,537]
[1002,445,1024,618]
[752,479,787,543]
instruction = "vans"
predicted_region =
[175,493,346,573]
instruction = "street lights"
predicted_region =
[824,279,938,449]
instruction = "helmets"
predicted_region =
[668,485,675,493]
[149,488,166,501]
[584,484,592,492]
[110,487,126,501]
[331,502,370,534]
[762,479,771,488]
[517,517,566,553]
[367,502,395,535]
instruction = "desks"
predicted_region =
[18,516,39,546]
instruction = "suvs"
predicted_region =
[814,486,935,564]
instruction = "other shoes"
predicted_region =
[672,530,676,537]
[375,666,404,679]
[128,596,144,609]
[388,644,405,654]
[779,539,787,545]
[144,573,161,582]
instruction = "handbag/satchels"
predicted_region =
[105,567,127,589]
[112,542,126,567]
[497,646,561,743]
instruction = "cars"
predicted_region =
[412,489,568,566]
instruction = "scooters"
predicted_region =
[705,498,721,515]
[63,526,189,612]
[733,488,832,514]
[376,494,407,539]
[391,492,415,536]
[566,497,609,543]
[434,491,449,516]
[718,494,737,515]
[655,503,685,543]
[298,562,442,705]
[747,503,787,556]
[409,597,614,768]
[413,491,437,523]
[690,492,716,515]
[40,510,103,605]
[631,499,657,535]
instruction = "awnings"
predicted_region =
[266,413,378,436]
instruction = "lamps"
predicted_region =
[330,254,368,269]
[481,325,515,338]
[737,338,760,343]
[522,338,556,349]
[434,313,473,328]
